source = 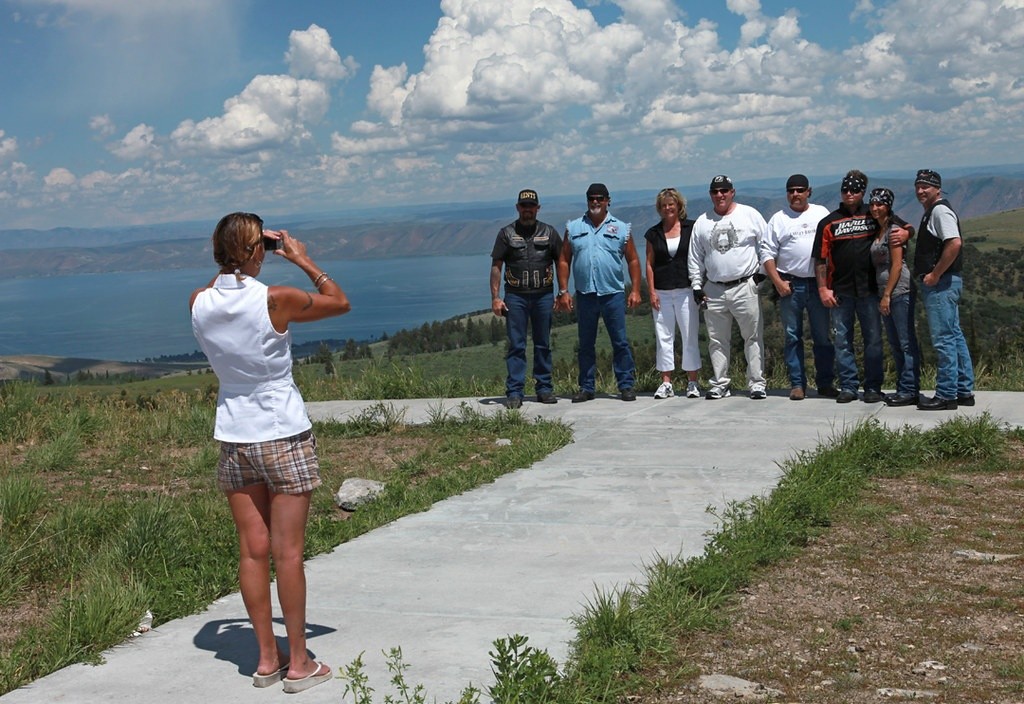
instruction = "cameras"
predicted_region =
[700,301,708,310]
[264,235,285,250]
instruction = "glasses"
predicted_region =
[661,188,678,193]
[917,169,937,178]
[840,186,862,194]
[710,188,730,194]
[786,187,808,193]
[587,197,606,201]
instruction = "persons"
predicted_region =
[869,188,922,407]
[558,183,641,403]
[644,188,702,399]
[811,170,915,404]
[490,189,564,409]
[188,212,352,693]
[913,170,977,409]
[687,175,770,400]
[760,175,838,399]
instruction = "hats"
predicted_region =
[517,188,539,205]
[914,171,942,188]
[710,175,734,189]
[587,183,609,198]
[786,173,809,188]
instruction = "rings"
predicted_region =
[883,309,887,311]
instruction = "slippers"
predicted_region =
[252,658,290,688]
[282,660,333,693]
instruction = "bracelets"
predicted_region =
[557,289,568,298]
[314,272,327,286]
[316,277,333,290]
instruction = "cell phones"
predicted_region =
[502,310,508,317]
[774,283,795,297]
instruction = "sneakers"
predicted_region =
[686,383,701,397]
[536,391,557,404]
[620,388,636,401]
[572,392,595,403]
[886,395,920,406]
[916,392,976,411]
[790,387,805,399]
[863,391,884,403]
[837,392,857,402]
[750,387,766,398]
[705,386,731,399]
[818,385,840,398]
[506,395,522,409]
[653,383,674,399]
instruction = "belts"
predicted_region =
[717,276,751,286]
